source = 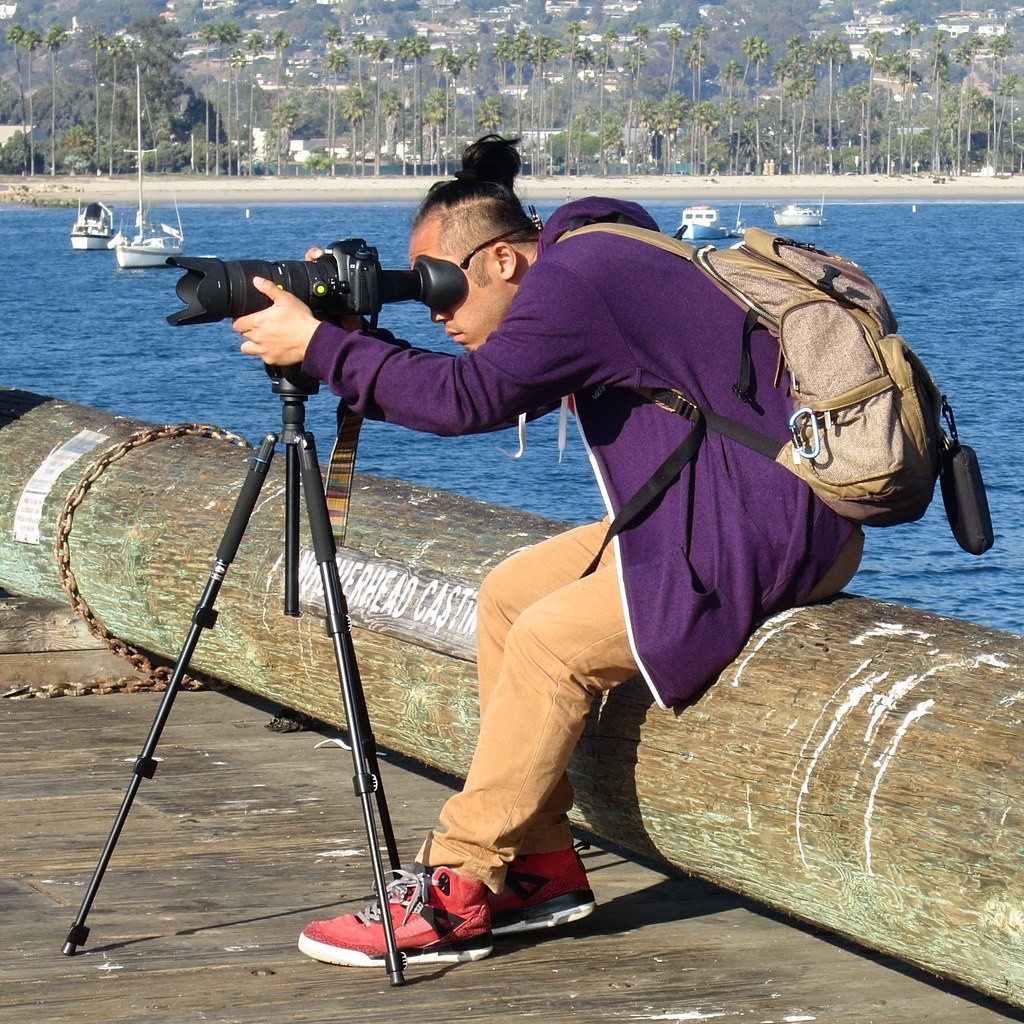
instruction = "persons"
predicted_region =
[231,134,865,965]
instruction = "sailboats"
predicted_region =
[114,64,186,267]
[729,202,749,238]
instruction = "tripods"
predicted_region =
[62,356,407,985]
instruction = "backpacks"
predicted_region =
[554,221,997,579]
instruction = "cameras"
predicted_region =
[165,236,383,325]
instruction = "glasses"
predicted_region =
[460,203,543,271]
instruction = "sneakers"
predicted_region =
[297,865,494,968]
[487,847,597,937]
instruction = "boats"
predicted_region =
[773,191,827,227]
[70,200,114,250]
[677,205,728,241]
